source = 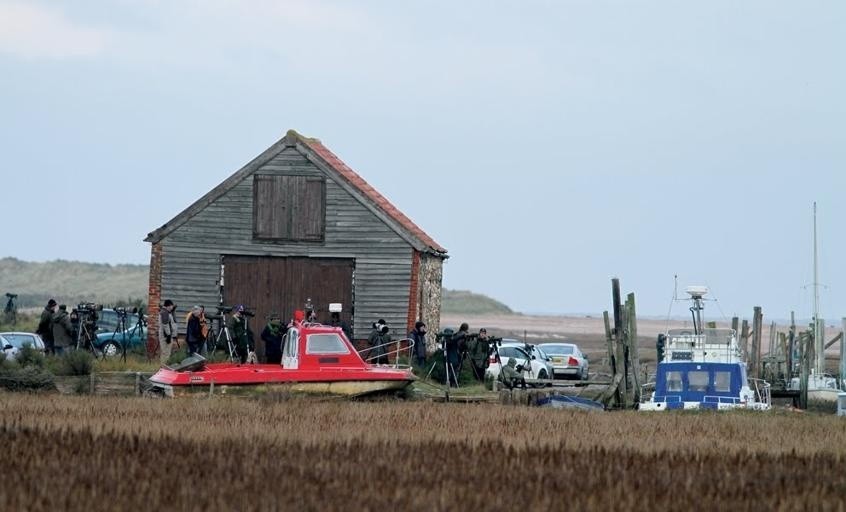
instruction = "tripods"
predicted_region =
[3,299,251,364]
[362,332,534,390]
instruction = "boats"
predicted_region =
[787,368,845,404]
[536,394,607,413]
[636,276,775,413]
[147,318,422,402]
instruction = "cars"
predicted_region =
[484,337,592,389]
[93,321,148,359]
[0,331,47,363]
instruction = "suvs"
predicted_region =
[93,308,148,337]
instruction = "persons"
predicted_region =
[186,304,208,357]
[496,356,523,384]
[226,303,252,363]
[184,303,206,358]
[366,318,392,364]
[406,320,426,368]
[34,298,79,356]
[153,297,179,366]
[441,322,489,384]
[284,310,352,341]
[259,312,287,363]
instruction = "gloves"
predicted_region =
[166,335,172,343]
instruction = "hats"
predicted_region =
[47,299,57,307]
[164,299,173,307]
